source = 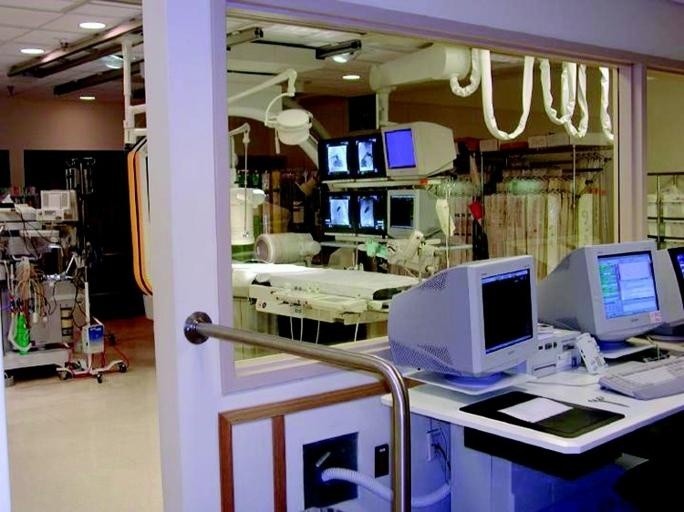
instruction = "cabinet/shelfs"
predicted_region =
[0,218,79,382]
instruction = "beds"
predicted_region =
[227,264,425,341]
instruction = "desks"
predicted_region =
[379,338,684,511]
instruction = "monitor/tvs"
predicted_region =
[354,193,382,231]
[649,247,684,344]
[322,138,353,180]
[387,187,440,240]
[537,239,666,363]
[385,253,538,397]
[382,122,458,180]
[326,190,355,232]
[351,132,383,179]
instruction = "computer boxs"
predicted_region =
[536,324,581,378]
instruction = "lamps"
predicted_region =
[227,67,315,145]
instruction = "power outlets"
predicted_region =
[427,429,443,462]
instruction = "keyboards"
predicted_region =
[599,357,684,402]
